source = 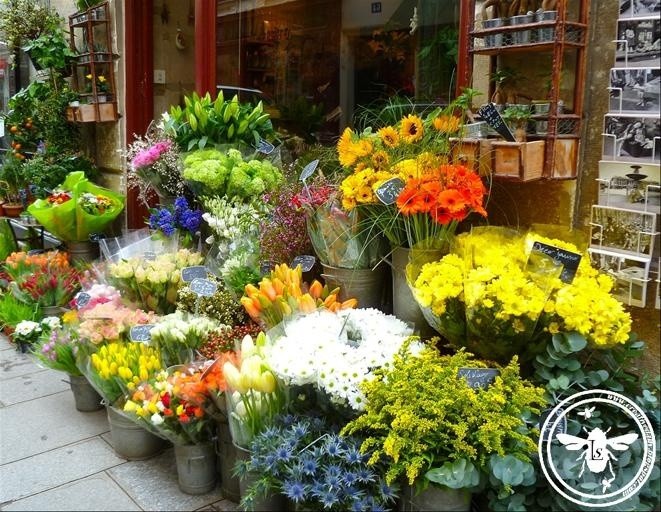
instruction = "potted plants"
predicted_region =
[0,293,35,344]
[21,23,77,77]
[2,148,25,218]
[0,0,60,71]
[68,89,80,107]
[491,106,546,184]
[338,335,547,512]
[78,44,107,61]
[535,67,573,135]
[442,87,487,140]
[74,0,105,23]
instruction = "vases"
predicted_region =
[40,303,60,318]
[214,421,242,503]
[320,261,387,313]
[233,440,278,512]
[101,399,161,460]
[175,440,217,494]
[86,92,107,104]
[66,239,95,264]
[61,374,103,414]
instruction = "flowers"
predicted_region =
[458,223,523,268]
[240,262,359,327]
[27,168,85,241]
[54,264,83,305]
[174,137,282,199]
[169,89,284,152]
[78,299,159,344]
[465,244,564,364]
[121,224,179,315]
[109,363,217,446]
[268,306,426,411]
[169,241,205,315]
[232,415,401,511]
[97,235,138,304]
[522,278,632,385]
[290,179,388,269]
[28,326,89,375]
[75,181,125,241]
[522,213,614,290]
[85,73,107,92]
[117,118,190,209]
[405,228,466,352]
[260,167,328,273]
[222,332,289,448]
[12,315,63,345]
[198,190,262,268]
[339,85,497,249]
[23,268,58,307]
[176,281,246,326]
[77,344,165,407]
[5,249,69,273]
[193,323,258,363]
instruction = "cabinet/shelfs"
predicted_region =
[66,0,119,124]
[452,0,592,185]
